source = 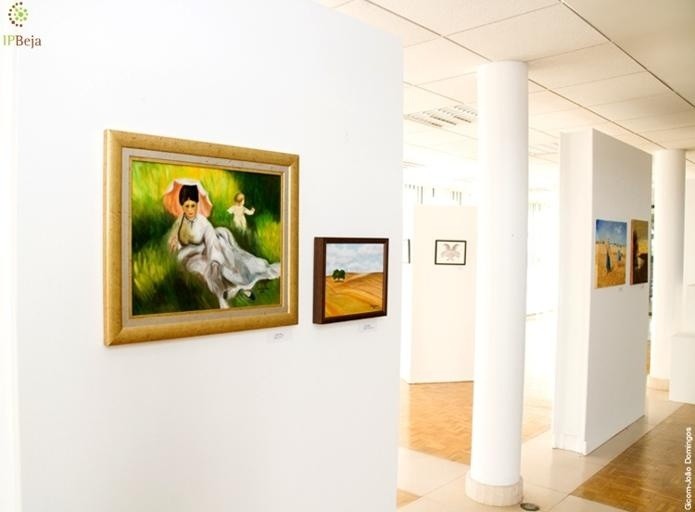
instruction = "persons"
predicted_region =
[168,185,281,310]
[226,193,256,229]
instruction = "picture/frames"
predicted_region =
[313,237,389,325]
[102,128,300,347]
[434,240,467,265]
